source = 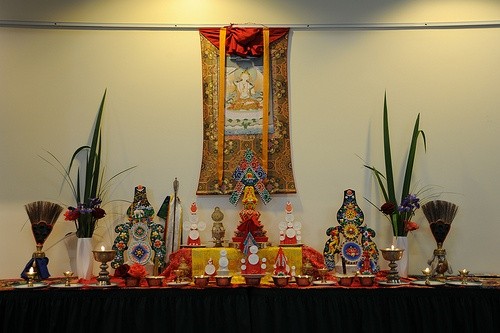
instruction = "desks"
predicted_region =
[0,275,500,333]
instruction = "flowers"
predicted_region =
[18,87,139,252]
[354,88,462,235]
[114,262,147,278]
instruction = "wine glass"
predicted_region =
[22,272,37,287]
[317,268,330,282]
[62,272,73,286]
[458,269,470,283]
[173,269,184,284]
[421,269,434,285]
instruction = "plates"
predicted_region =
[167,282,189,285]
[313,282,335,284]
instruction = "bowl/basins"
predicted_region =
[338,273,356,285]
[216,275,233,287]
[146,276,165,286]
[195,275,209,286]
[357,275,376,286]
[294,276,313,286]
[272,275,291,285]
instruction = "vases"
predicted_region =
[393,236,409,278]
[76,238,93,279]
